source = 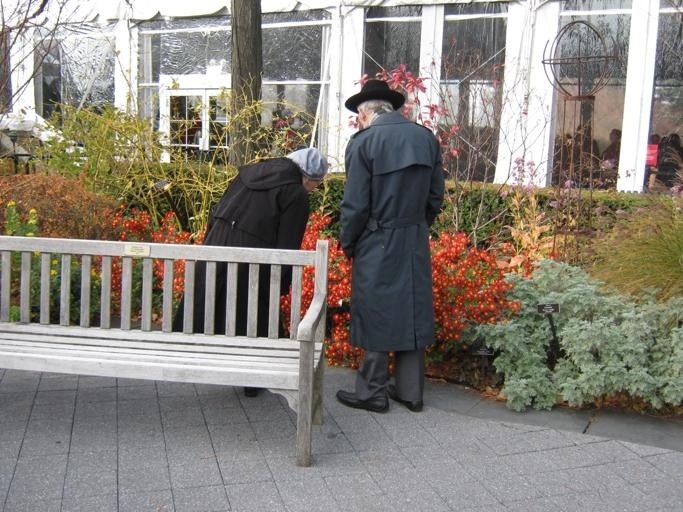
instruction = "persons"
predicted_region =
[171,147,328,398]
[552,124,683,196]
[335,79,445,414]
[274,108,297,151]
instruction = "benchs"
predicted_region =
[0,235,329,467]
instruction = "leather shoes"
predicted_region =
[334,389,389,414]
[387,384,423,412]
[243,387,261,397]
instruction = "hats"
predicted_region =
[344,79,404,114]
[282,147,328,182]
